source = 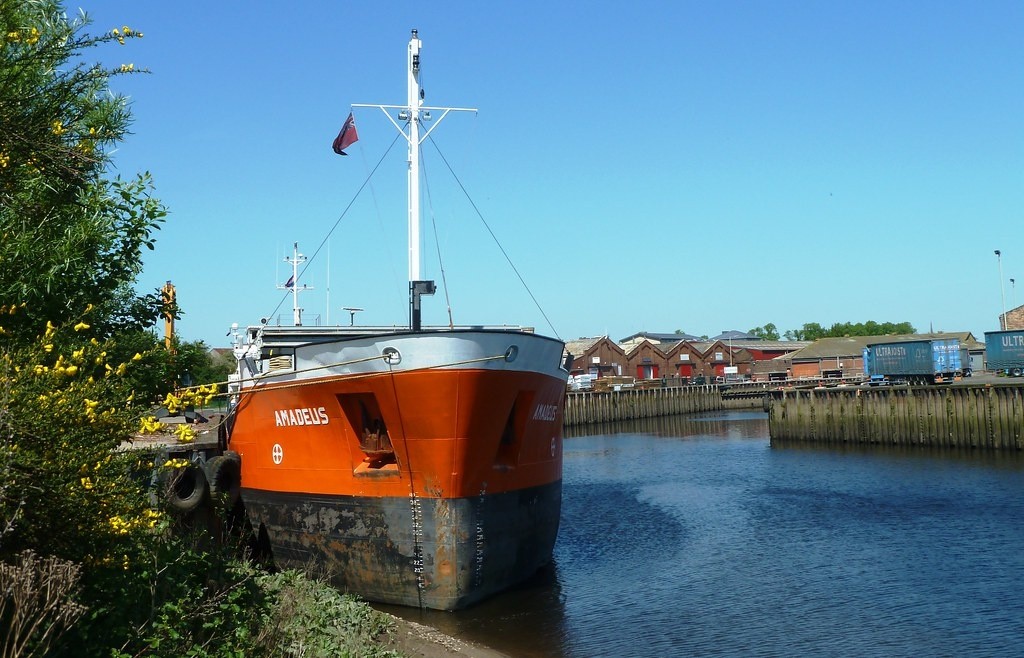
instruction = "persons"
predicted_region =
[697,374,704,384]
[661,374,668,387]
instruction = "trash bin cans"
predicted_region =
[662,378,667,387]
[697,376,705,385]
[682,376,688,386]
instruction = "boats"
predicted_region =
[223,28,573,613]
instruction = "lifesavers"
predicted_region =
[158,456,240,511]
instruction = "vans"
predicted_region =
[567,373,597,390]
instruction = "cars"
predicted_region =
[689,377,714,384]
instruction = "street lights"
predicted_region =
[1011,278,1017,307]
[995,249,1008,330]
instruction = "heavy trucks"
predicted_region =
[983,329,1024,377]
[862,337,973,386]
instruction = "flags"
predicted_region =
[332,111,359,155]
